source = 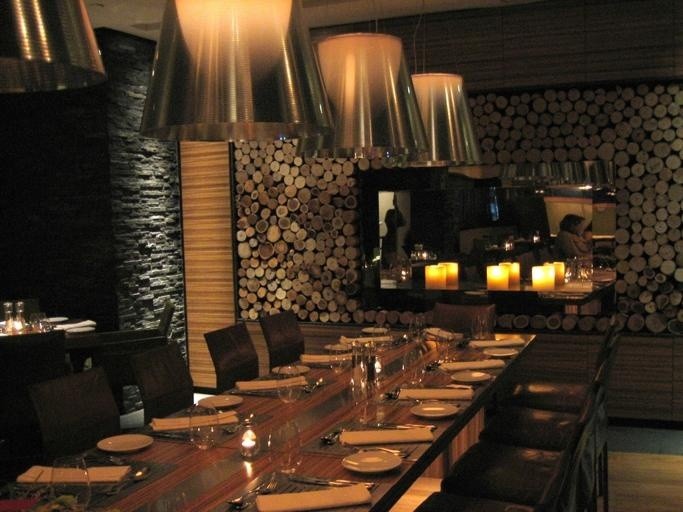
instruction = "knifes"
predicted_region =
[364,422,437,431]
[288,475,375,489]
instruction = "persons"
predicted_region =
[553,213,589,262]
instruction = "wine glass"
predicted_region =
[188,406,222,463]
[349,366,376,425]
[408,315,427,348]
[49,455,91,512]
[266,419,304,492]
[434,327,456,365]
[402,349,426,406]
[275,365,302,413]
[329,344,352,379]
[29,312,47,332]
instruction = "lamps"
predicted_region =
[139,0,336,142]
[488,190,499,222]
[295,0,429,158]
[0,0,104,94]
[500,161,614,190]
[393,0,483,166]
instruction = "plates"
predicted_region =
[95,434,154,453]
[362,327,388,334]
[323,344,352,351]
[271,365,310,375]
[341,450,403,474]
[483,349,519,357]
[450,371,492,383]
[198,394,243,409]
[409,403,460,418]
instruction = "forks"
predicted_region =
[239,480,278,512]
[352,446,418,459]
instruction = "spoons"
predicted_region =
[318,429,340,444]
[225,482,264,504]
[304,377,327,393]
[106,466,152,495]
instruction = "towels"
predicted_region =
[467,337,527,348]
[148,409,239,433]
[337,334,395,344]
[435,359,508,372]
[337,424,437,447]
[396,385,475,402]
[15,464,134,486]
[252,484,374,512]
[422,326,463,342]
[234,374,308,391]
[297,350,354,365]
[54,318,98,331]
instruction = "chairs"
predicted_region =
[259,310,305,373]
[24,367,123,463]
[0,297,41,321]
[516,252,537,282]
[477,353,618,512]
[415,457,572,511]
[67,301,175,371]
[204,323,259,394]
[507,315,625,412]
[432,302,496,333]
[129,343,194,425]
[440,391,600,512]
[0,330,67,426]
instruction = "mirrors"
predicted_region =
[359,160,617,291]
